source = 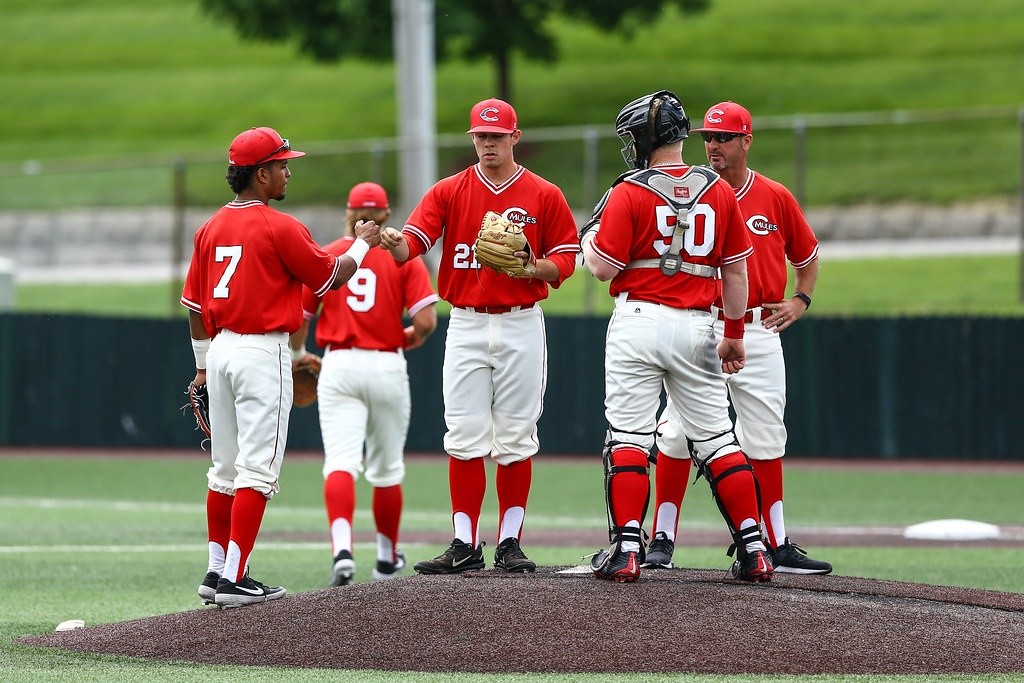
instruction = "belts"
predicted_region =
[626,295,713,314]
[718,307,774,325]
[452,302,535,315]
[330,343,398,353]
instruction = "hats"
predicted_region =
[465,97,518,134]
[227,126,305,167]
[691,101,753,135]
[348,182,389,209]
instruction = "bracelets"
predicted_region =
[290,344,306,362]
[792,292,811,311]
[343,238,370,271]
[724,315,746,339]
[191,337,211,369]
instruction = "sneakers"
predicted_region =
[327,549,355,588]
[591,550,641,582]
[732,551,774,583]
[493,537,536,573]
[413,537,486,575]
[198,569,288,609]
[373,553,405,579]
[639,531,676,570]
[765,536,833,575]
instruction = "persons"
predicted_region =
[578,89,774,583]
[288,181,438,586]
[638,100,832,574]
[180,126,382,609]
[380,98,581,574]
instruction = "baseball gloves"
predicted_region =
[190,383,211,441]
[474,210,537,279]
[292,352,322,408]
[578,219,601,253]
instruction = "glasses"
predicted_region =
[256,138,290,163]
[700,132,746,143]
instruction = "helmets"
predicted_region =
[616,89,690,170]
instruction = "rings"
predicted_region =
[780,317,784,323]
